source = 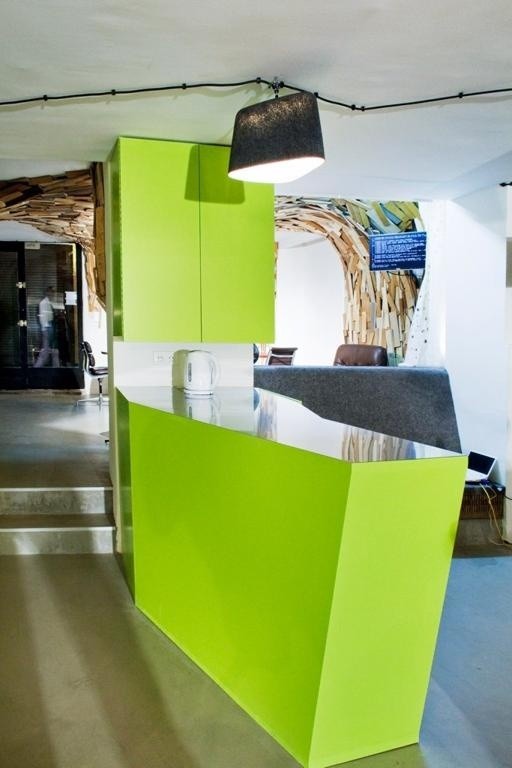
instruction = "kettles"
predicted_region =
[183,350,218,395]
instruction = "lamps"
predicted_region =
[223,88,325,184]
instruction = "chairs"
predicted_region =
[335,344,390,366]
[266,347,299,365]
[76,341,111,409]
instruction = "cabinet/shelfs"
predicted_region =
[112,138,277,346]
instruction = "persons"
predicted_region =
[34,287,60,368]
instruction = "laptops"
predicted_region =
[465,449,497,483]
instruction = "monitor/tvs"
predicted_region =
[368,231,427,271]
